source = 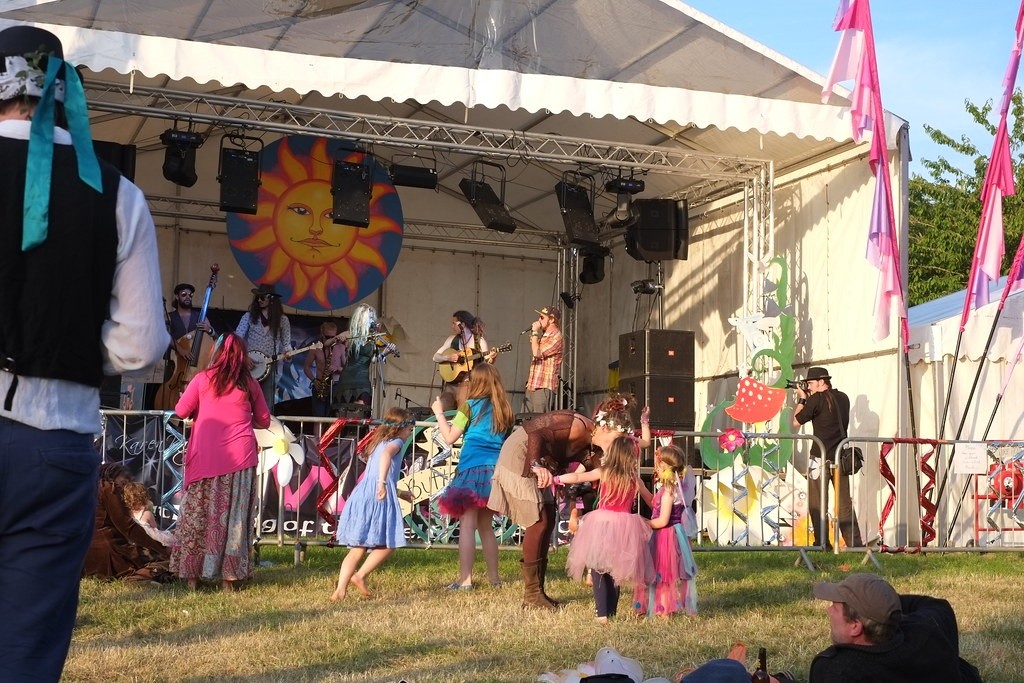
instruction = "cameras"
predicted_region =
[785,379,809,392]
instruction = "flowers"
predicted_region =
[253,415,305,487]
[719,429,745,452]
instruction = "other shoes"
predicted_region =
[223,581,246,590]
[447,582,472,590]
[185,581,200,590]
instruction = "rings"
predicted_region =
[542,473,544,476]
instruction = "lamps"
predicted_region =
[574,244,611,284]
[216,127,264,216]
[560,291,582,308]
[331,141,376,229]
[459,158,517,234]
[629,279,666,295]
[159,116,205,187]
[605,167,646,230]
[387,152,440,195]
[555,162,602,248]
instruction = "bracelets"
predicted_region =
[377,479,387,485]
[556,476,560,484]
[208,328,213,334]
[380,353,386,359]
[312,378,315,382]
[798,398,805,405]
[398,490,402,497]
[436,412,444,419]
[532,330,539,336]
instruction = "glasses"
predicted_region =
[540,316,545,319]
[180,291,194,298]
[323,333,334,339]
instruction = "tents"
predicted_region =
[907,275,1024,552]
[0,0,913,554]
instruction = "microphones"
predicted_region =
[520,326,532,335]
[370,320,378,328]
[456,321,464,332]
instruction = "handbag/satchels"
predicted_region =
[841,447,864,475]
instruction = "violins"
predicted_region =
[369,324,401,358]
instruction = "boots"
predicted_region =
[519,557,556,610]
[539,559,558,607]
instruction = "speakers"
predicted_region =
[618,329,695,379]
[626,199,689,262]
[630,434,696,520]
[618,378,694,428]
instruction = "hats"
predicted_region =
[800,367,832,381]
[0,26,103,250]
[251,283,282,298]
[534,306,560,320]
[813,574,902,624]
[174,283,195,293]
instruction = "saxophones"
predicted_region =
[316,342,334,402]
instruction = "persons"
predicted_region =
[751,573,981,683]
[487,394,699,625]
[0,26,171,683]
[431,363,515,589]
[521,306,564,413]
[163,282,396,441]
[85,331,271,593]
[793,367,863,549]
[332,407,416,600]
[433,310,497,420]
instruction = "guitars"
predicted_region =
[247,341,323,383]
[439,340,513,384]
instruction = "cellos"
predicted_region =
[154,263,221,411]
